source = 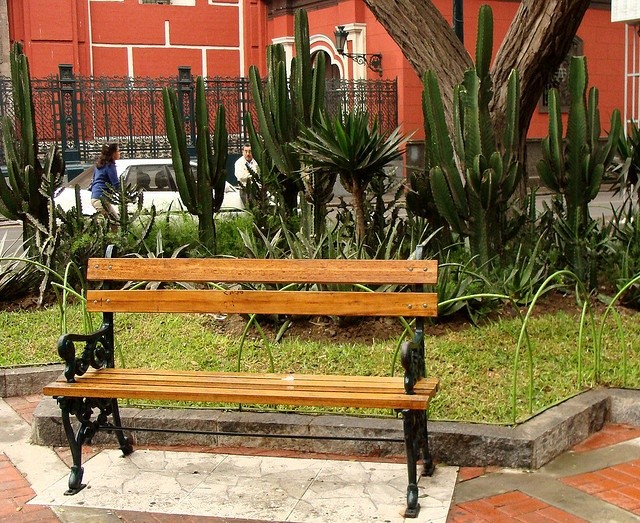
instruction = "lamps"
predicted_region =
[332,24,349,54]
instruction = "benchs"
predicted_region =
[41,243,441,519]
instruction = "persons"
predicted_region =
[90,141,121,233]
[234,143,262,210]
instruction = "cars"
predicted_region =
[41,158,303,227]
